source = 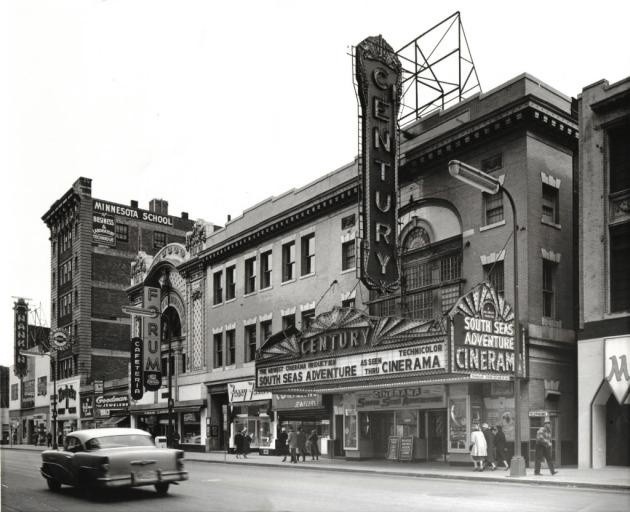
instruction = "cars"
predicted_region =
[40,428,189,502]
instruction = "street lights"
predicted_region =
[18,349,58,452]
[120,304,173,452]
[446,159,528,478]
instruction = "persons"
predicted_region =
[47,432,52,447]
[234,431,252,458]
[172,430,180,449]
[534,422,558,476]
[13,432,17,445]
[279,426,321,463]
[469,423,510,472]
